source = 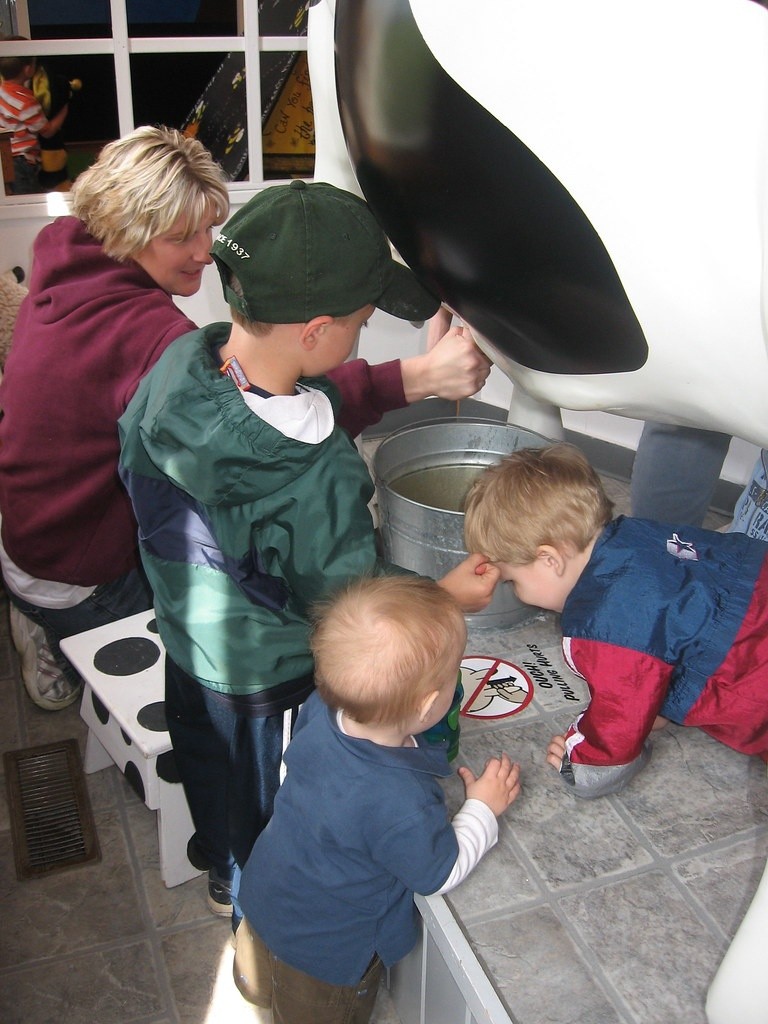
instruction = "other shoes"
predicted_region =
[231,906,245,950]
[207,867,234,917]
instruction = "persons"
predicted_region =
[233,573,522,1024]
[0,36,74,196]
[464,446,768,798]
[117,178,441,918]
[0,126,491,710]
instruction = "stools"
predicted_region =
[57,607,209,888]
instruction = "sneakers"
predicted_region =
[10,601,86,710]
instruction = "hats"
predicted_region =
[208,179,442,322]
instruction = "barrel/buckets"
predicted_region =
[373,416,574,630]
